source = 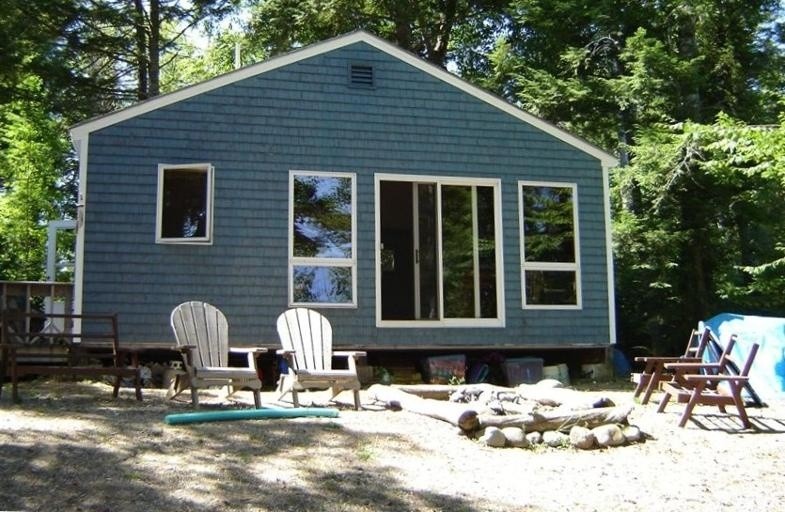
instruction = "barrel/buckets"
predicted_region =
[542,363,571,385]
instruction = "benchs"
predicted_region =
[0,311,143,403]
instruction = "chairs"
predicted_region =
[170,299,366,412]
[631,327,759,427]
